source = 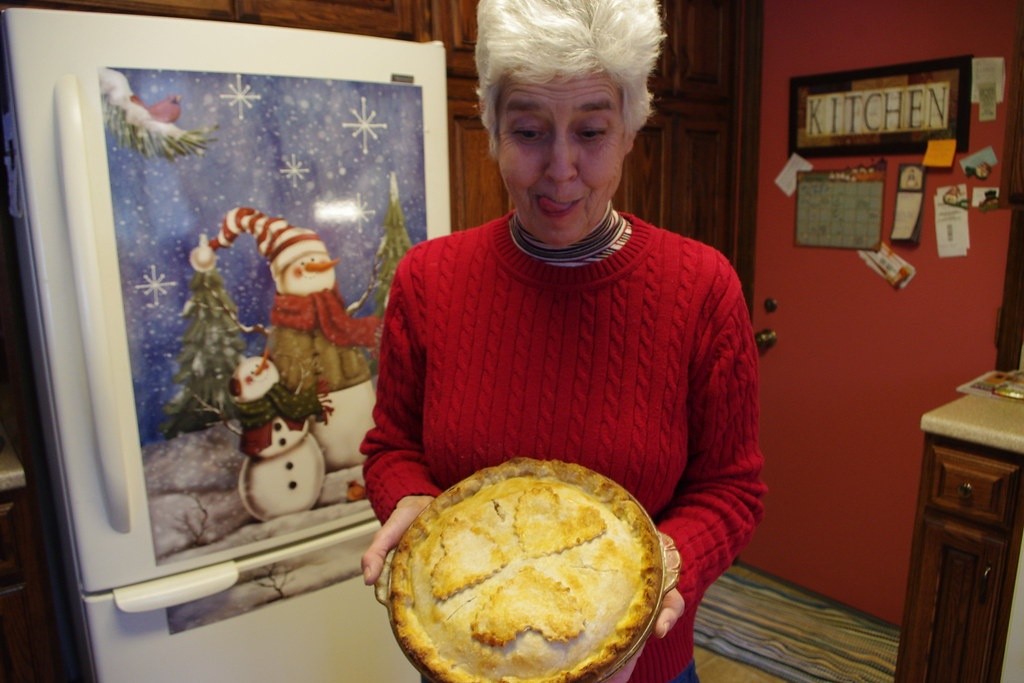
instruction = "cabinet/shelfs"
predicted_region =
[891,362,1024,683]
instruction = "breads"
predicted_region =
[388,458,663,683]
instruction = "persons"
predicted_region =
[358,0,770,683]
